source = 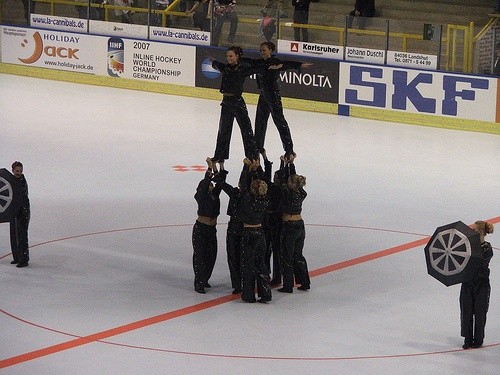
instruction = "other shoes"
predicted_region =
[11,260,28,267]
[278,287,293,293]
[298,284,310,290]
[462,336,483,349]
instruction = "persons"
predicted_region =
[460,221,494,349]
[212,148,310,303]
[240,42,313,158]
[191,156,229,294]
[75,0,139,23]
[22,0,36,26]
[292,0,311,42]
[347,0,375,30]
[9,161,31,268]
[155,0,238,45]
[493,43,500,76]
[208,46,283,164]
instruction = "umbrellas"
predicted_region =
[0,168,24,223]
[424,220,484,287]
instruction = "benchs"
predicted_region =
[218,0,497,68]
